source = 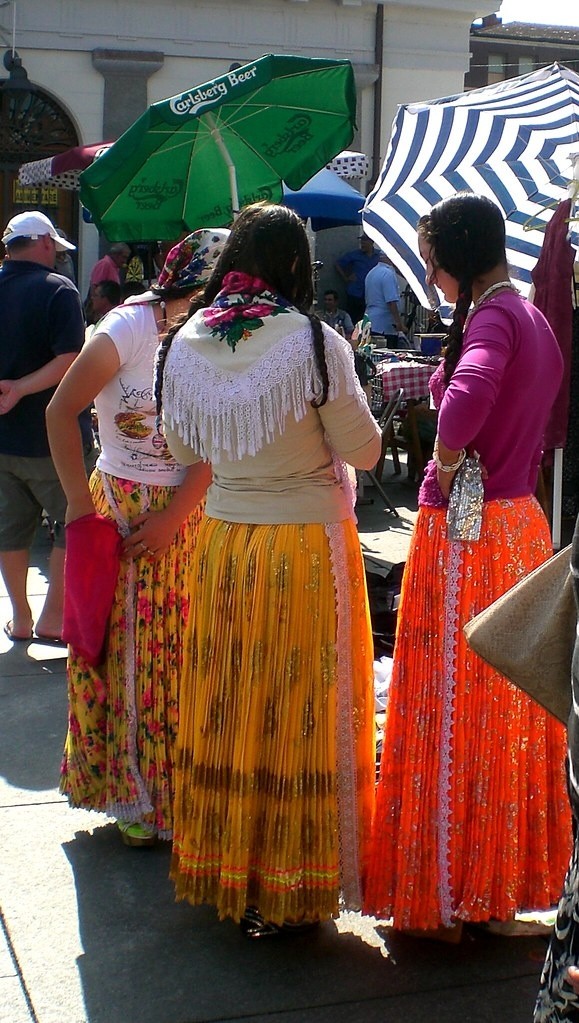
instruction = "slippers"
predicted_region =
[4,620,32,641]
[32,637,65,644]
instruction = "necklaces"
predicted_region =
[462,281,522,333]
[314,290,355,339]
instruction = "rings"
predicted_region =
[140,542,147,550]
[147,549,154,556]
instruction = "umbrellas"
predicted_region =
[358,61,579,334]
[280,167,366,232]
[325,150,369,179]
[77,52,359,242]
[17,141,115,191]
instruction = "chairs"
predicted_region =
[351,388,403,518]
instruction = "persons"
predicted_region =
[54,229,146,315]
[361,191,574,944]
[0,209,97,643]
[333,232,406,328]
[152,199,383,928]
[45,227,232,847]
[361,250,403,349]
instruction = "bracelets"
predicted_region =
[432,433,466,472]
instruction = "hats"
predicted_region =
[56,229,67,240]
[1,211,76,251]
[357,234,374,243]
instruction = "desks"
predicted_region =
[369,351,441,486]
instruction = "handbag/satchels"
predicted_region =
[61,513,123,667]
[447,459,484,541]
[462,543,577,725]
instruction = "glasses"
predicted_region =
[93,292,102,297]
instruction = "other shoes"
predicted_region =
[241,907,320,937]
[117,818,154,846]
